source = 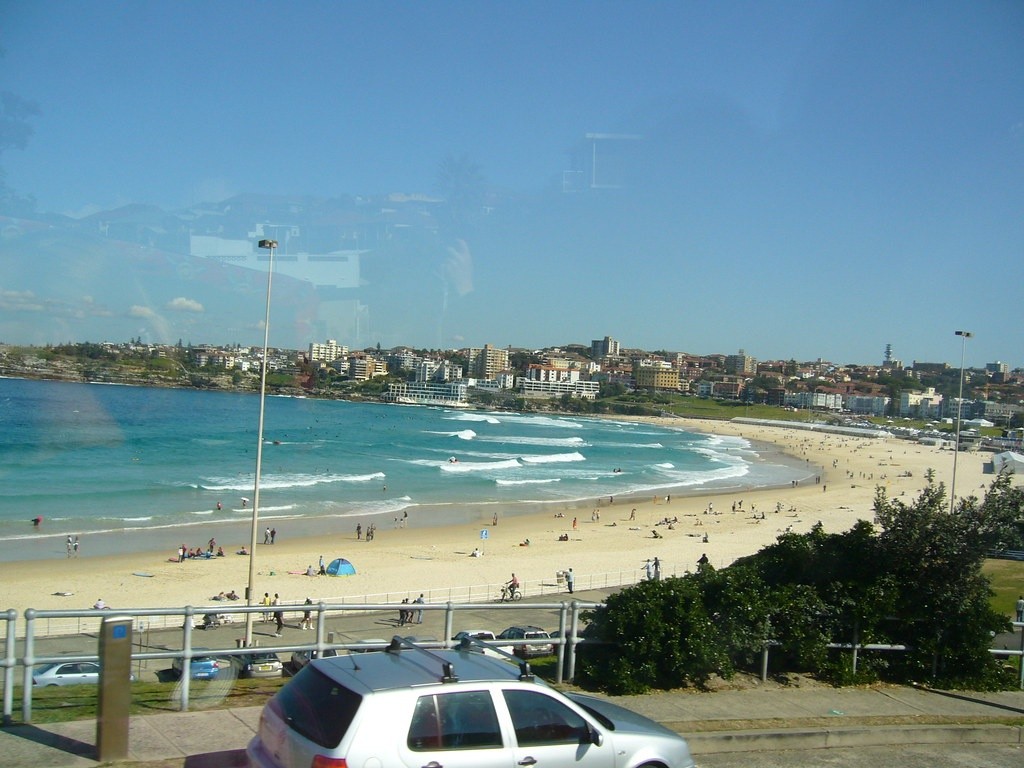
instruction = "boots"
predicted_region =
[310,622,314,629]
[302,623,307,629]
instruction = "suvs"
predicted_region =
[246,634,694,768]
[290,649,338,671]
[497,624,553,655]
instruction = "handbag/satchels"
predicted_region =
[516,583,519,588]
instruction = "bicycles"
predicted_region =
[500,584,522,602]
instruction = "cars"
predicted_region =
[231,653,283,679]
[451,630,496,655]
[171,647,219,680]
[347,639,387,654]
[403,635,441,649]
[550,630,584,652]
[32,662,133,686]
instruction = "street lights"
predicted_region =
[244,238,278,646]
[950,330,973,513]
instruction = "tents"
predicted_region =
[326,558,356,574]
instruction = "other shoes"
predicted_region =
[274,633,282,637]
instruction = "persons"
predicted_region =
[317,556,325,576]
[616,468,621,471]
[505,573,518,598]
[306,565,313,576]
[775,429,913,495]
[652,557,660,580]
[65,535,72,554]
[74,537,80,553]
[697,553,708,565]
[469,548,480,558]
[564,568,573,594]
[178,500,276,563]
[493,495,798,546]
[355,484,408,543]
[640,559,651,581]
[93,591,428,638]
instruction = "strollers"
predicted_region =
[203,614,218,631]
